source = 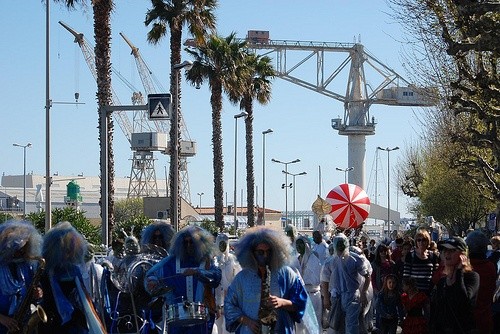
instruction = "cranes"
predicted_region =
[57,20,195,200]
[182,26,440,188]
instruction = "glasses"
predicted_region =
[416,238,427,242]
[255,248,274,256]
[184,239,194,244]
[153,233,163,240]
[380,248,388,253]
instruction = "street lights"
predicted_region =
[272,158,307,232]
[338,165,354,190]
[262,125,273,228]
[231,109,247,238]
[10,141,34,218]
[378,143,401,236]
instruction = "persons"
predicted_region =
[0,215,500,334]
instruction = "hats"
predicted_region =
[438,235,468,252]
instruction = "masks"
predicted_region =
[186,246,195,255]
[154,238,164,245]
[256,254,271,266]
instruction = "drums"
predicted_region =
[166,301,206,327]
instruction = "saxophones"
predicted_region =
[7,254,48,334]
[258,265,277,334]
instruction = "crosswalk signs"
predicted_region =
[146,93,172,121]
